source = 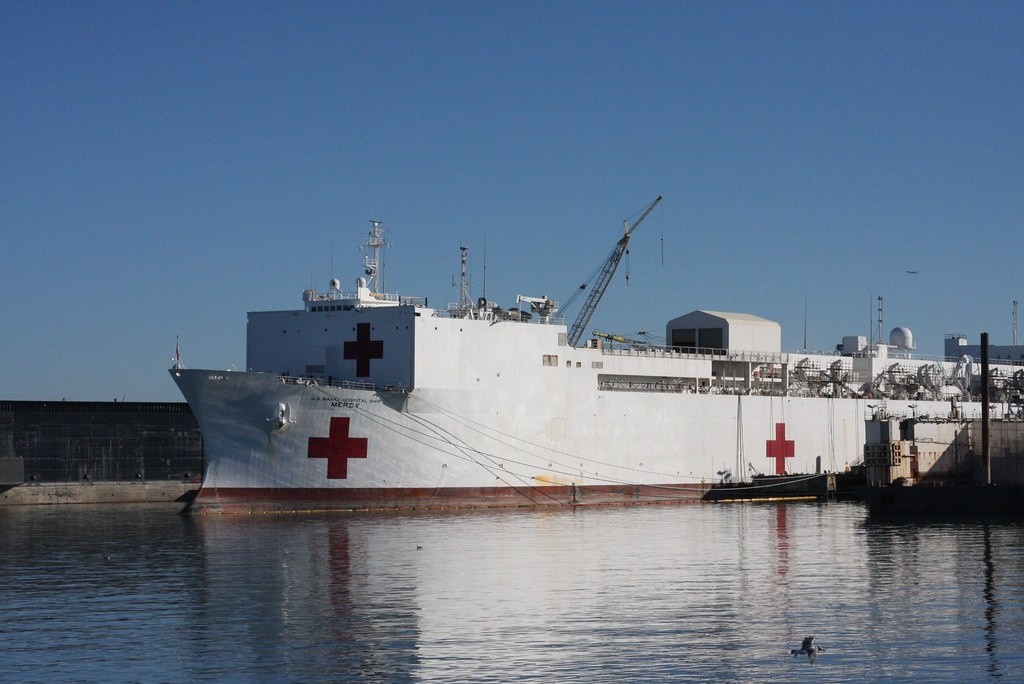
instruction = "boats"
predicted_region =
[166,195,1023,519]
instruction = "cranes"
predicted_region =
[563,196,664,347]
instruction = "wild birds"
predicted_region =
[789,636,826,668]
